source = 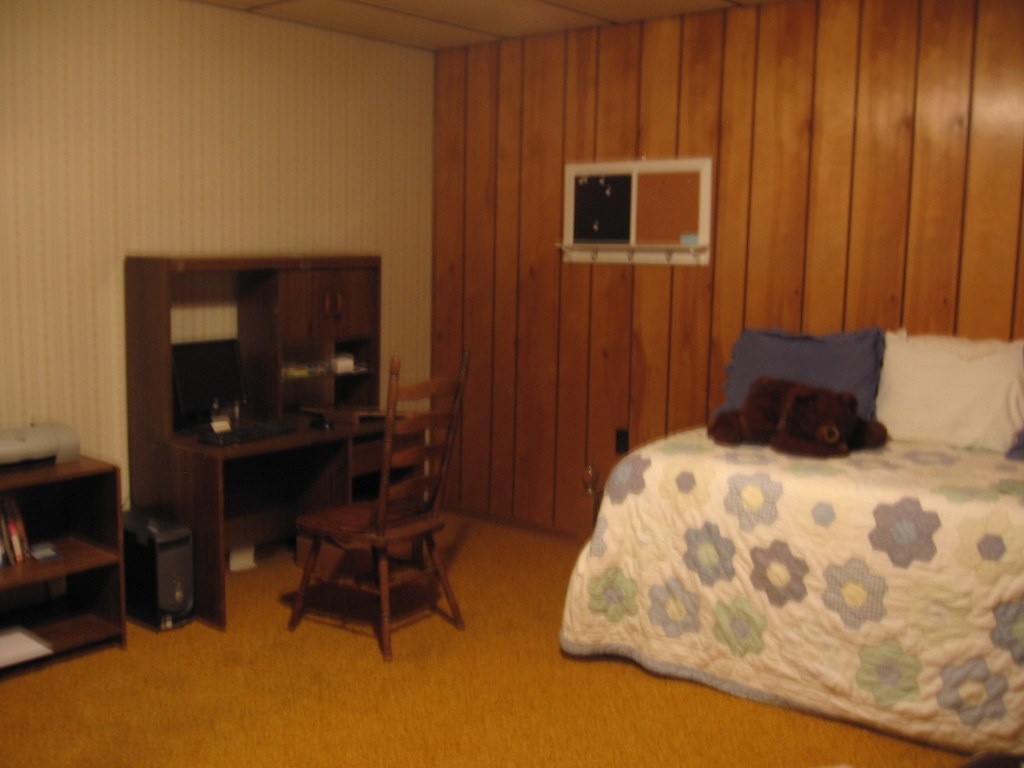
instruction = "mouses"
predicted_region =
[310,414,329,431]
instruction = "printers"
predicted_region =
[0,422,83,474]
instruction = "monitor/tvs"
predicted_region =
[172,339,249,425]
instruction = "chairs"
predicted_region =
[286,349,473,660]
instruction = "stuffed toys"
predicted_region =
[708,373,889,459]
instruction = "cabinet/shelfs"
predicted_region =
[0,453,128,677]
[124,251,427,438]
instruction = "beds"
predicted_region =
[557,428,1024,760]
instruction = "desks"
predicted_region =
[130,409,427,631]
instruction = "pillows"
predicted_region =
[874,327,1024,455]
[710,327,884,424]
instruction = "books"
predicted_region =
[0,496,30,567]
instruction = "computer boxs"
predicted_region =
[123,509,194,628]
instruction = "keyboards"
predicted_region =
[198,421,296,446]
